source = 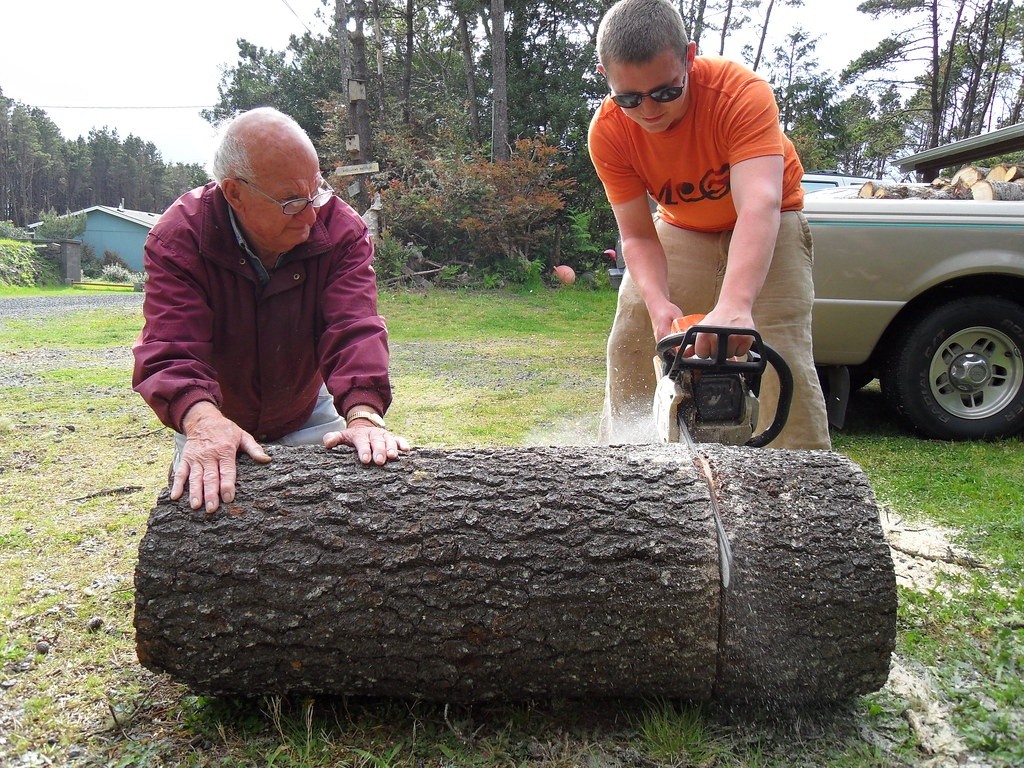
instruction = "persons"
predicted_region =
[133,105,412,513]
[589,1,832,452]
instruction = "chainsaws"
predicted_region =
[651,313,794,592]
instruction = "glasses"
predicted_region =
[609,45,688,108]
[235,176,335,216]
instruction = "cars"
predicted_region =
[800,174,900,200]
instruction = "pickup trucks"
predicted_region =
[803,119,1024,442]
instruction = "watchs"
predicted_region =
[347,412,386,428]
